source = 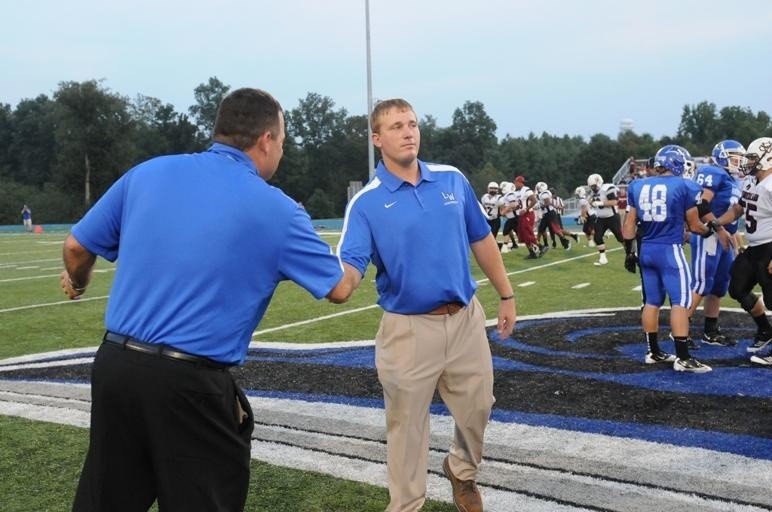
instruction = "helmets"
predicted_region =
[652,145,695,180]
[711,140,747,177]
[738,137,772,176]
[500,181,517,195]
[535,182,547,195]
[587,173,604,194]
[488,181,499,196]
[574,187,586,200]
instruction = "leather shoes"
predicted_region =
[442,454,485,512]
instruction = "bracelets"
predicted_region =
[500,294,514,300]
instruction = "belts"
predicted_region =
[424,300,467,318]
[99,330,226,373]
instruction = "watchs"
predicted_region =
[67,278,88,292]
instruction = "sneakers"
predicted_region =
[668,328,703,349]
[538,247,548,261]
[750,351,772,368]
[644,348,676,364]
[524,254,537,260]
[702,331,737,347]
[588,240,595,248]
[748,331,772,353]
[563,240,572,252]
[674,358,712,374]
[575,234,580,244]
[593,258,609,266]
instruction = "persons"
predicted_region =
[514,176,549,259]
[21,204,33,231]
[535,181,580,251]
[574,185,609,248]
[549,187,565,248]
[535,187,549,248]
[669,140,746,350]
[622,157,657,228]
[621,144,713,374]
[704,136,772,367]
[327,98,518,512]
[59,86,354,512]
[481,181,503,252]
[586,173,626,266]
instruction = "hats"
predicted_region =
[513,176,528,183]
[645,157,655,168]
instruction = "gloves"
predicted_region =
[577,217,587,226]
[702,221,717,239]
[591,201,604,207]
[622,238,639,274]
